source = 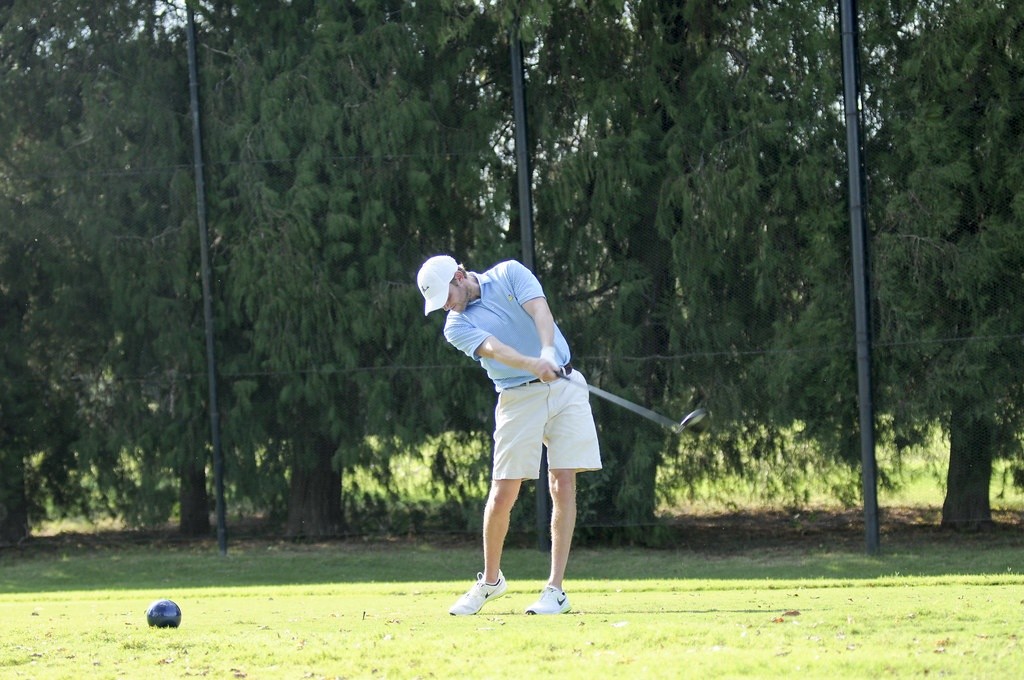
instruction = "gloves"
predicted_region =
[541,346,561,373]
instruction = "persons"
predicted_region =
[417,255,602,616]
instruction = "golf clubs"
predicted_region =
[554,370,710,434]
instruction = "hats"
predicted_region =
[417,255,457,316]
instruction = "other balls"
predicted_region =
[148,600,183,629]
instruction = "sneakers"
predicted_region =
[525,583,572,616]
[450,568,506,615]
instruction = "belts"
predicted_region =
[524,363,572,385]
